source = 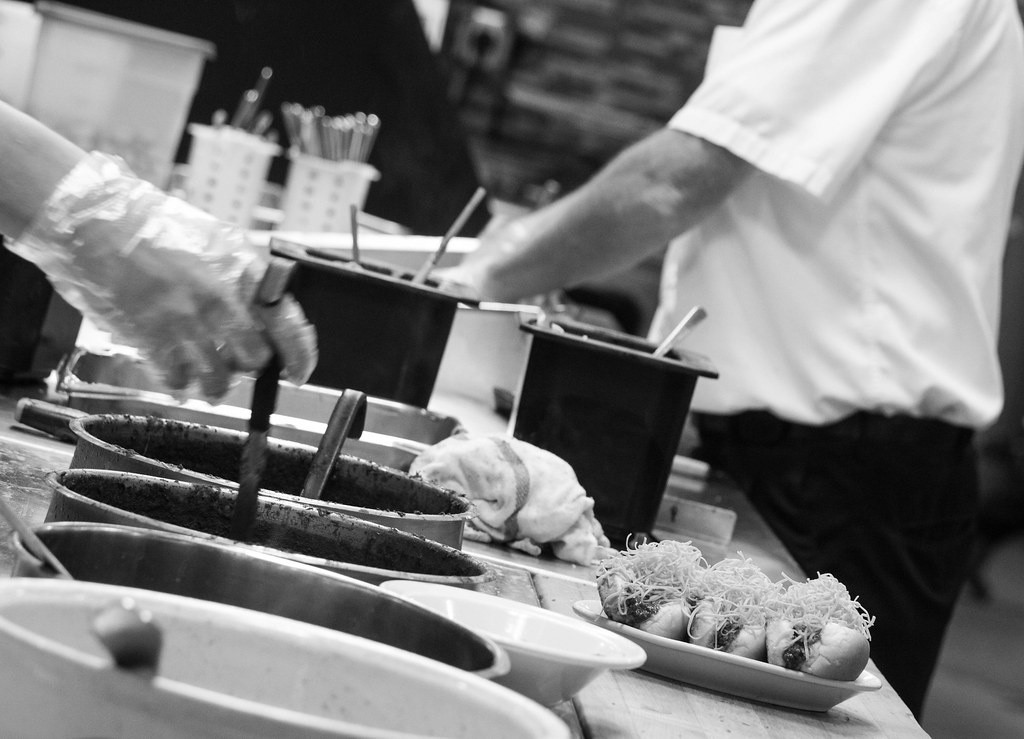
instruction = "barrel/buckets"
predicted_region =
[513,317,719,546]
[268,236,480,411]
[24,2,214,190]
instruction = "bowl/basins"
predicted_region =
[379,580,647,708]
[43,469,499,587]
[70,415,478,552]
[12,522,510,682]
[1,577,570,739]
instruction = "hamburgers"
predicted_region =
[599,569,870,683]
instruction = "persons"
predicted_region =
[0,99,319,401]
[435,0,1024,726]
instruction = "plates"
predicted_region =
[573,600,882,710]
